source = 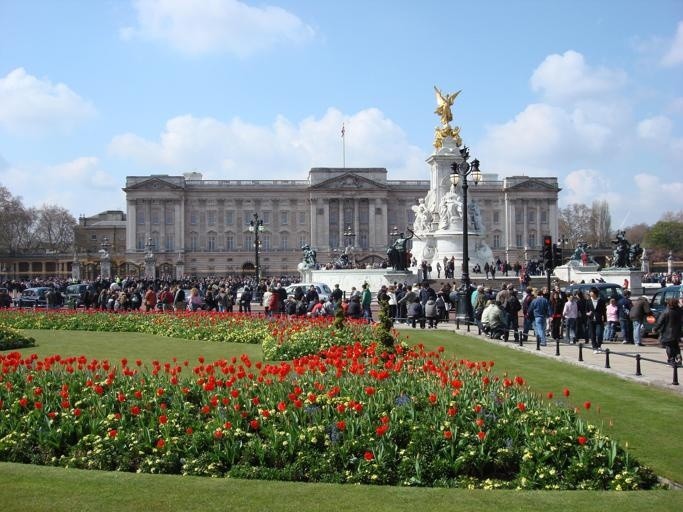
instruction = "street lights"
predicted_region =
[450,145,480,326]
[557,235,568,265]
[251,212,262,283]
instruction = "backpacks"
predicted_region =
[472,306,487,320]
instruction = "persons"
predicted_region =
[611,231,642,266]
[391,230,415,257]
[565,241,591,260]
[0,255,683,367]
[432,85,462,147]
[300,243,316,263]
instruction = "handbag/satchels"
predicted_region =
[215,293,222,300]
[445,303,452,311]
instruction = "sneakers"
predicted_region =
[593,348,602,354]
[492,335,508,342]
[622,340,645,347]
[666,359,682,367]
[428,324,438,329]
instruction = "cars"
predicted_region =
[0,288,13,308]
[639,285,683,337]
[284,284,332,308]
[14,284,97,308]
[564,283,623,303]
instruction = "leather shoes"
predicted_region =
[514,338,528,342]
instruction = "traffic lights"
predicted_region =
[543,236,553,270]
